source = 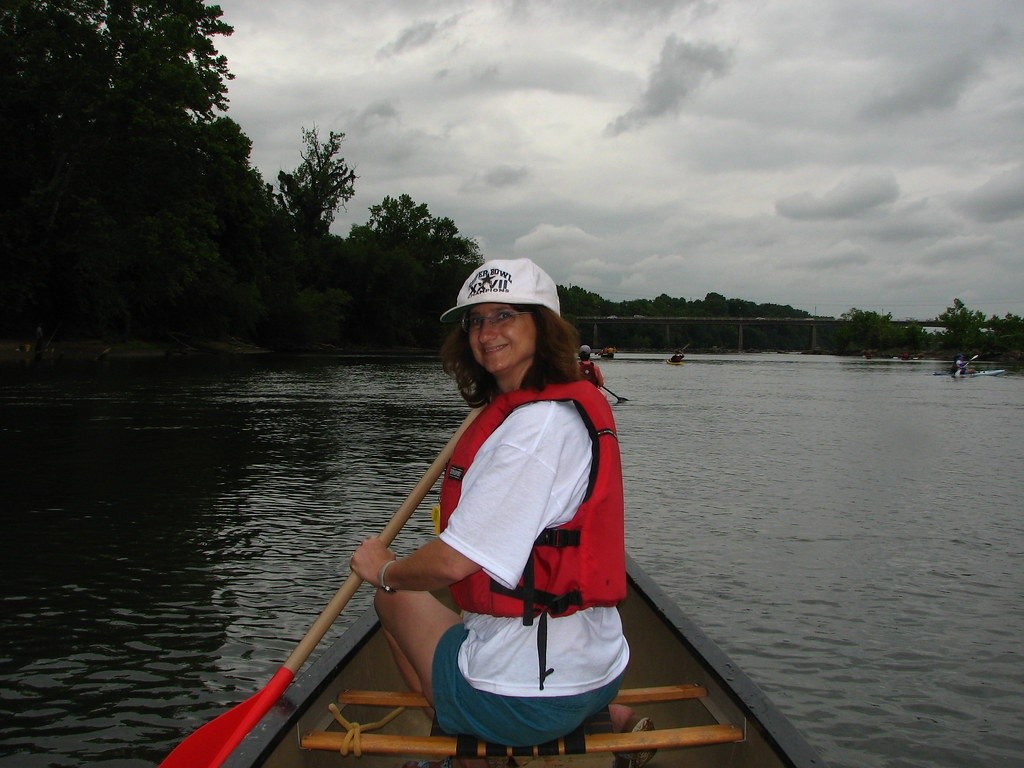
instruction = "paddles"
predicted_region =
[954,354,979,378]
[157,398,483,768]
[680,343,690,352]
[602,386,629,403]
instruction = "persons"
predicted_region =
[595,345,617,355]
[349,257,658,768]
[864,348,873,359]
[577,345,605,388]
[950,353,976,374]
[900,347,910,360]
[669,348,684,362]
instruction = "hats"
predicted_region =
[439,258,561,323]
[578,344,591,356]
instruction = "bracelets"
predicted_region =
[379,559,398,593]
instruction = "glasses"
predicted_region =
[462,312,534,333]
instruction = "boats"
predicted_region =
[777,350,789,354]
[666,359,688,366]
[579,351,591,361]
[596,351,614,359]
[928,368,1006,379]
[801,344,838,355]
[898,352,924,360]
[863,350,894,359]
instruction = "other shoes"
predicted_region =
[405,756,460,768]
[612,714,657,768]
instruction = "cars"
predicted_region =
[607,315,618,320]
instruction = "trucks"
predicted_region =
[634,314,647,319]
